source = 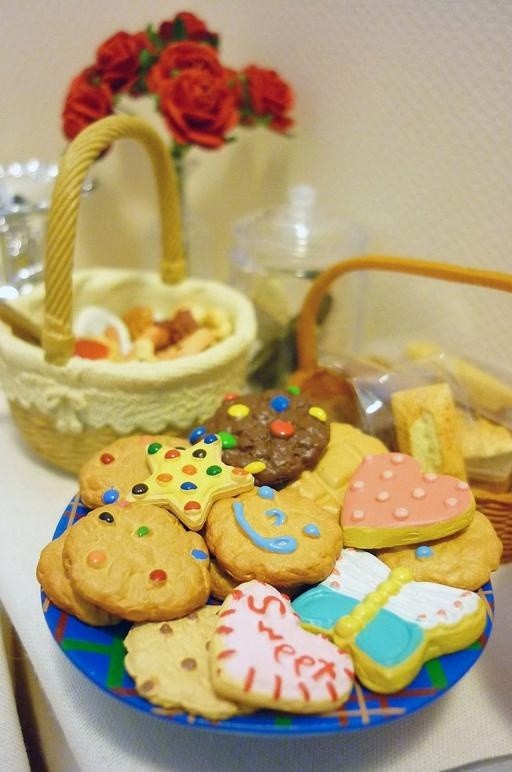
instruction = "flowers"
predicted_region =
[64,12,296,263]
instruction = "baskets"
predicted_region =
[284,255,512,566]
[0,114,258,476]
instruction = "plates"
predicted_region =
[40,495,496,737]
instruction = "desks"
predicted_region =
[0,369,511,772]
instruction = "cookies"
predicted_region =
[67,304,216,361]
[35,339,512,725]
